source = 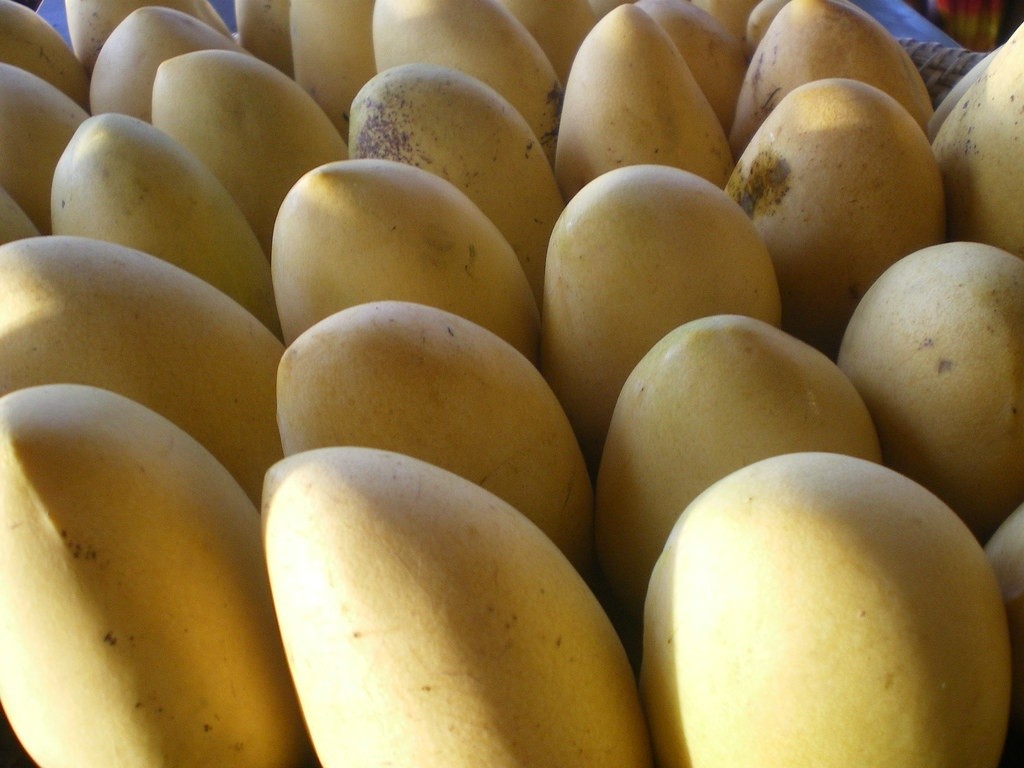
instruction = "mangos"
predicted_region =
[0,0,1024,768]
